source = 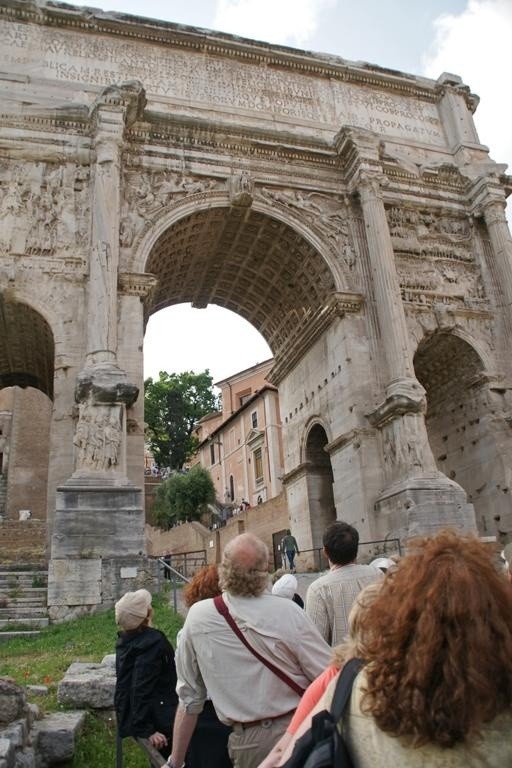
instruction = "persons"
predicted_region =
[269,189,355,264]
[257,495,262,504]
[114,522,403,768]
[70,411,119,471]
[120,170,208,247]
[500,542,512,569]
[278,527,512,768]
[211,498,249,530]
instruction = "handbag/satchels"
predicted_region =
[275,660,366,768]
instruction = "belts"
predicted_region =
[241,713,288,727]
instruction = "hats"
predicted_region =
[369,558,395,570]
[114,589,153,631]
[272,574,298,600]
